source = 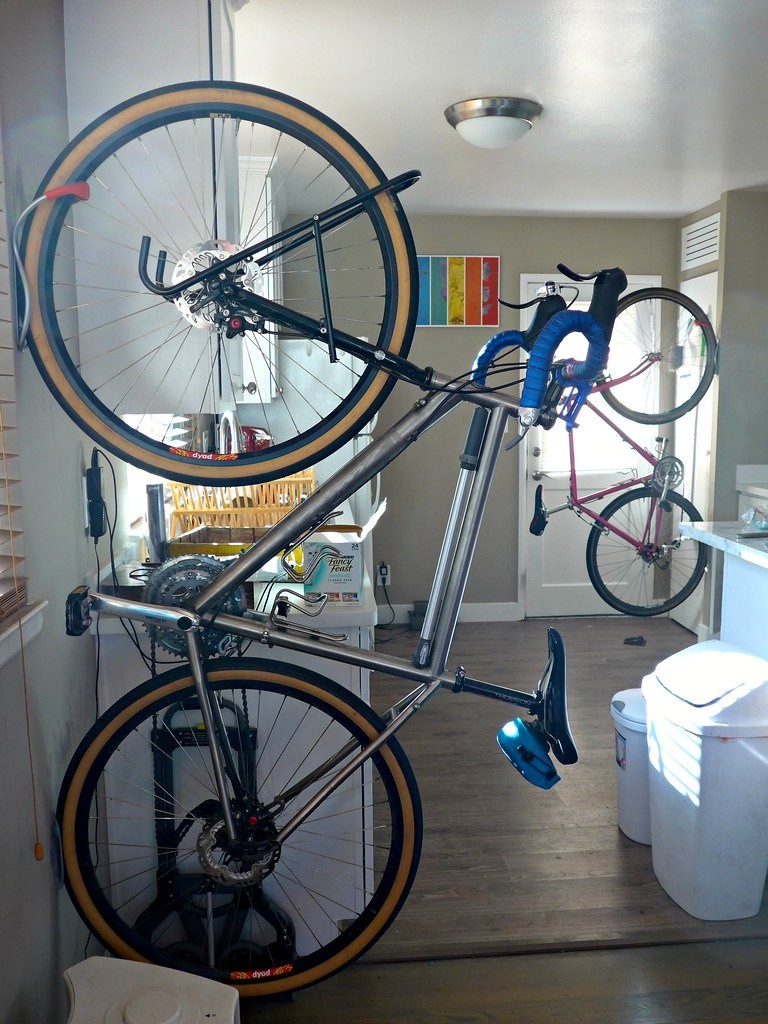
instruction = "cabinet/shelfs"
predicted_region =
[233,155,286,404]
[64,0,257,414]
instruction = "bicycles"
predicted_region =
[526,264,717,617]
[18,79,629,997]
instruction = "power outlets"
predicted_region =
[376,565,390,585]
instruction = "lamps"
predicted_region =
[444,95,541,151]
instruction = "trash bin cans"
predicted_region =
[609,687,650,848]
[643,637,768,923]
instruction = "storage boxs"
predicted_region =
[165,524,304,579]
[299,500,390,606]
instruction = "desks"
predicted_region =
[90,580,379,976]
